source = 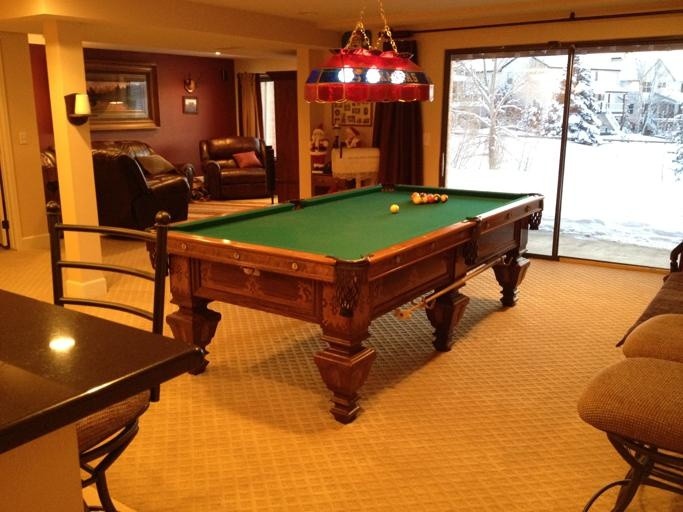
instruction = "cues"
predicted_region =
[399,248,529,317]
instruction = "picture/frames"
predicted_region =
[84,59,161,131]
[330,102,372,127]
[182,95,199,115]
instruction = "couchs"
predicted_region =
[38,140,194,234]
[199,136,276,201]
[91,148,190,237]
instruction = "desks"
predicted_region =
[0,287,202,512]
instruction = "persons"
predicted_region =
[310,116,328,175]
[341,126,363,148]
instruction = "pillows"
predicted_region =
[135,154,175,176]
[233,151,261,168]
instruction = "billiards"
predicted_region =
[441,194,448,202]
[428,194,433,196]
[420,193,425,196]
[428,196,433,202]
[412,192,418,197]
[421,196,428,203]
[391,204,399,213]
[434,194,440,201]
[413,196,421,204]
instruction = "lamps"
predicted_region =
[303,0,434,104]
[64,92,90,126]
[184,78,194,93]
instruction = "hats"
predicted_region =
[313,123,324,134]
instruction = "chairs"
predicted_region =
[575,357,682,512]
[44,201,171,512]
[622,313,682,363]
